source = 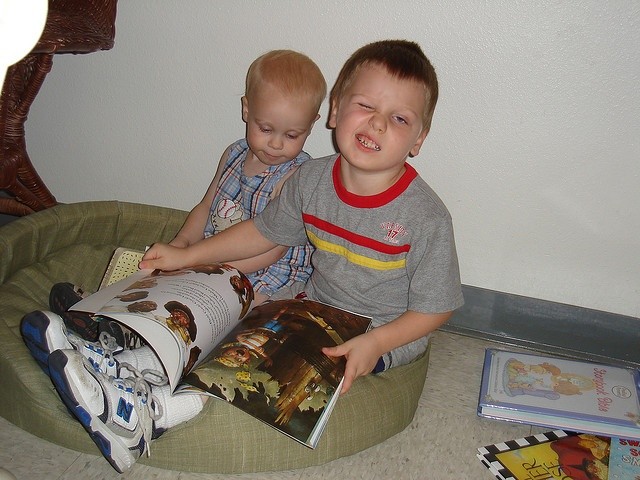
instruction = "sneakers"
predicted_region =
[50,281,90,312]
[19,308,120,395]
[48,348,168,472]
[61,311,124,348]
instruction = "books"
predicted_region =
[67,261,373,451]
[477,348,640,442]
[476,429,611,480]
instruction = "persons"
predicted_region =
[19,37,466,473]
[48,49,315,353]
